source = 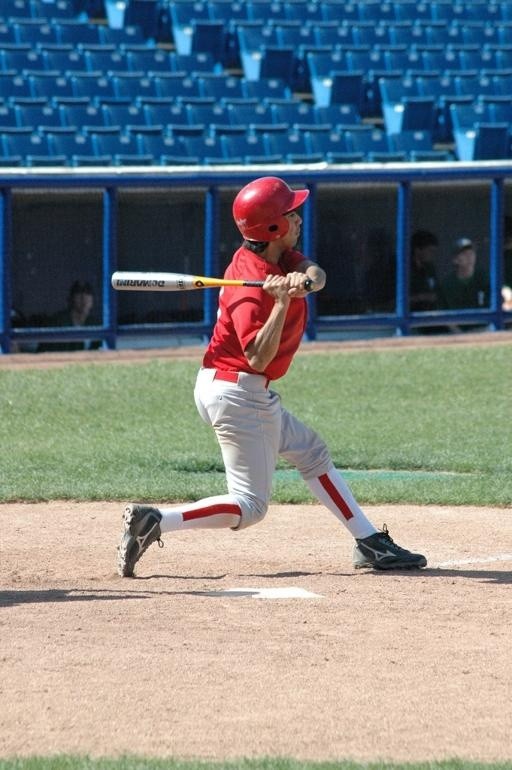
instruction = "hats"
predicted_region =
[450,237,479,257]
[232,175,311,243]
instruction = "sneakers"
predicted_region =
[116,503,164,580]
[351,530,427,572]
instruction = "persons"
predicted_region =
[425,237,511,335]
[112,174,429,579]
[373,229,439,318]
[36,275,102,353]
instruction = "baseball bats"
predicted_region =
[111,271,314,293]
